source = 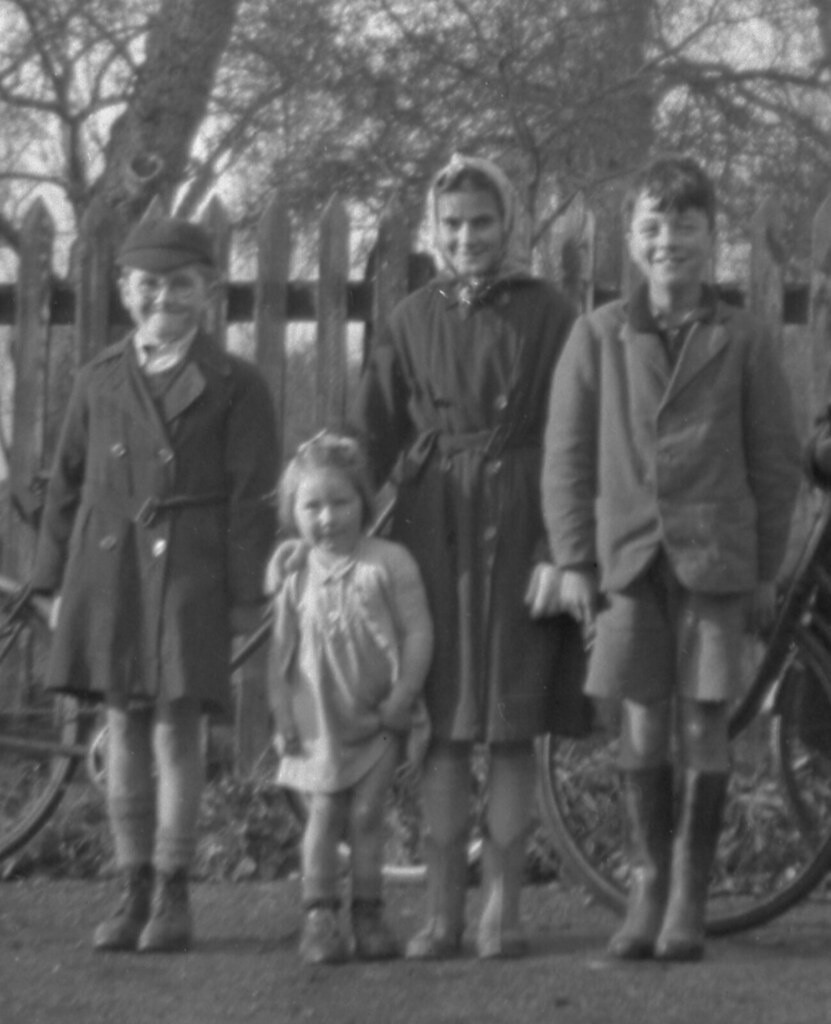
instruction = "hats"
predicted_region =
[113,218,215,271]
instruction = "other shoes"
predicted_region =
[352,907,397,957]
[298,908,346,963]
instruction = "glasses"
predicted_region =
[129,272,201,299]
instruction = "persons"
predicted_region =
[266,433,434,962]
[539,157,795,960]
[343,153,578,957]
[33,218,276,953]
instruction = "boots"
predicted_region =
[93,859,152,949]
[135,865,192,950]
[474,801,542,959]
[608,762,671,957]
[404,813,477,959]
[654,762,732,959]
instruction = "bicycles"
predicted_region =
[0,477,534,883]
[524,432,831,942]
